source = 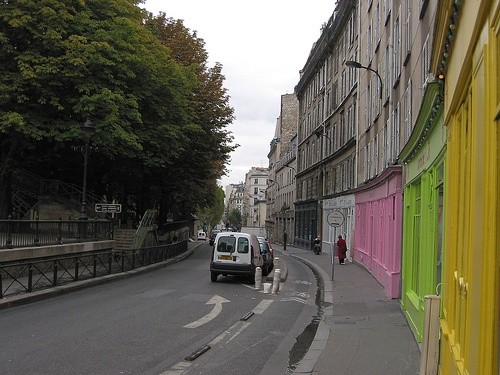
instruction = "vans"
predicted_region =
[210,232,264,284]
[198,232,207,241]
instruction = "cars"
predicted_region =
[256,236,266,244]
[210,233,218,246]
[258,242,274,275]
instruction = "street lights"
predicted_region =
[311,130,331,142]
[76,117,95,240]
[344,60,382,100]
[283,164,296,172]
[269,179,279,191]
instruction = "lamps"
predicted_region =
[310,130,332,155]
[345,60,383,99]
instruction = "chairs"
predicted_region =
[239,241,247,253]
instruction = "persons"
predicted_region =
[283,231,287,250]
[314,236,321,255]
[337,235,347,265]
